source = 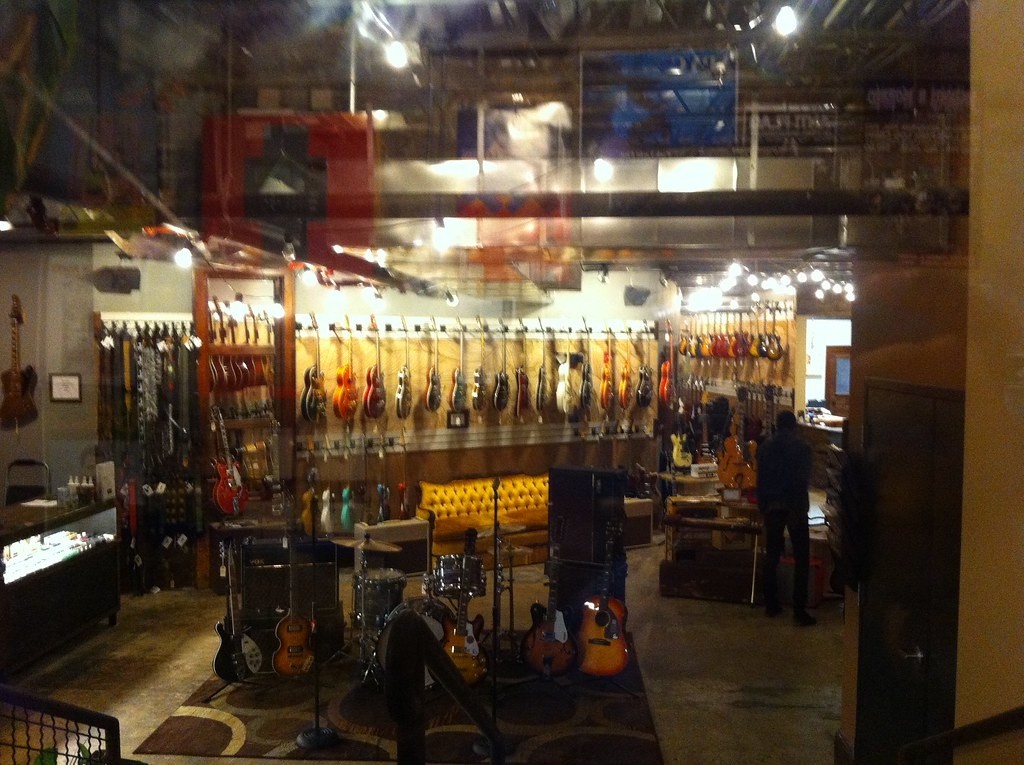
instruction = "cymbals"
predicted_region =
[330,536,403,553]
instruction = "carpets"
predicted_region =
[132,626,667,765]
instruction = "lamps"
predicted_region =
[92,265,142,295]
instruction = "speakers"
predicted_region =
[241,538,338,616]
[546,464,631,627]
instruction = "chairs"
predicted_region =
[3,457,51,508]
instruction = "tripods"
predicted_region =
[317,551,378,671]
[203,679,271,704]
[478,541,639,701]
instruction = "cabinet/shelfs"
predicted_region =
[664,493,723,562]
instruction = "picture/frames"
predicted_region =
[48,371,83,405]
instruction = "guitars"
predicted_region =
[300,424,410,538]
[679,309,784,360]
[1,293,40,431]
[209,294,277,392]
[575,516,635,678]
[271,527,317,678]
[440,526,487,693]
[212,537,263,684]
[519,514,580,678]
[669,371,778,489]
[210,403,250,516]
[300,322,676,419]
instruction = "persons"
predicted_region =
[755,410,818,625]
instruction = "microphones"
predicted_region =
[308,468,318,483]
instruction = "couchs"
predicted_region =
[414,467,654,572]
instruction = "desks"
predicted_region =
[661,468,724,497]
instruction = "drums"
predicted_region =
[374,595,482,701]
[435,553,487,598]
[353,566,407,629]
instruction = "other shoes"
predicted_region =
[792,611,817,626]
[765,606,786,616]
